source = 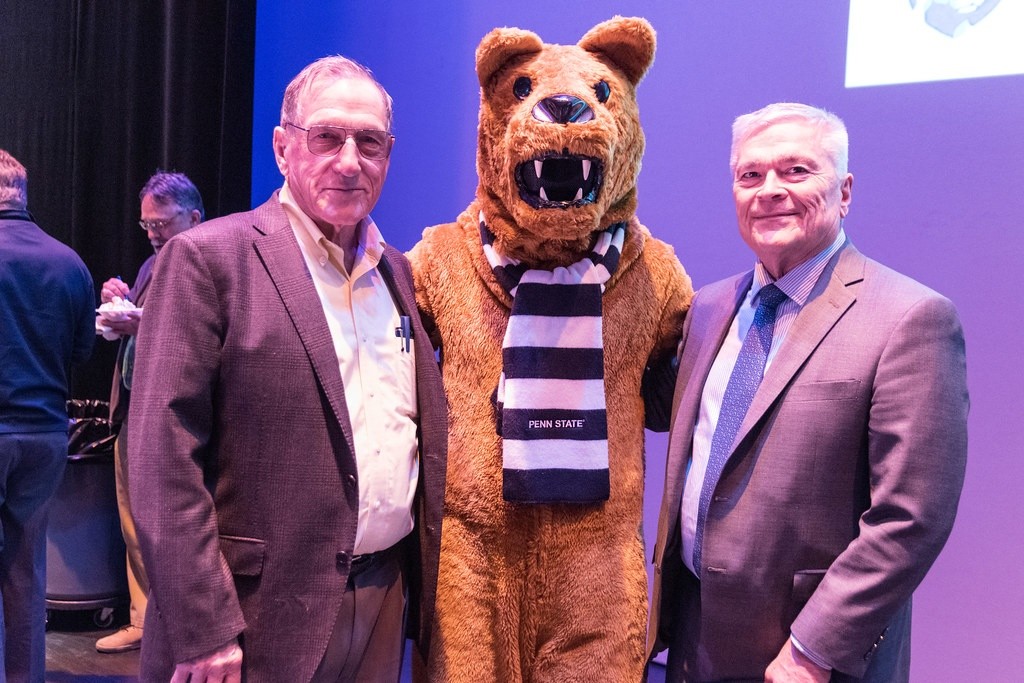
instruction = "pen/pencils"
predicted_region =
[400,315,411,353]
[395,326,404,354]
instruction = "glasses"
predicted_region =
[284,122,396,161]
[138,213,180,230]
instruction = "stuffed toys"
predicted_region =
[404,12,694,683]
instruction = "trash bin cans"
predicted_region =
[46,399,128,612]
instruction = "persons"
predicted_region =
[95,173,206,652]
[643,102,973,683]
[0,147,98,683]
[119,54,450,681]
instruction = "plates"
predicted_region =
[94,309,145,323]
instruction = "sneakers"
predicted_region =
[95,624,144,653]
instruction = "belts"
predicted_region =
[350,538,404,579]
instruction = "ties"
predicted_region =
[693,284,788,579]
